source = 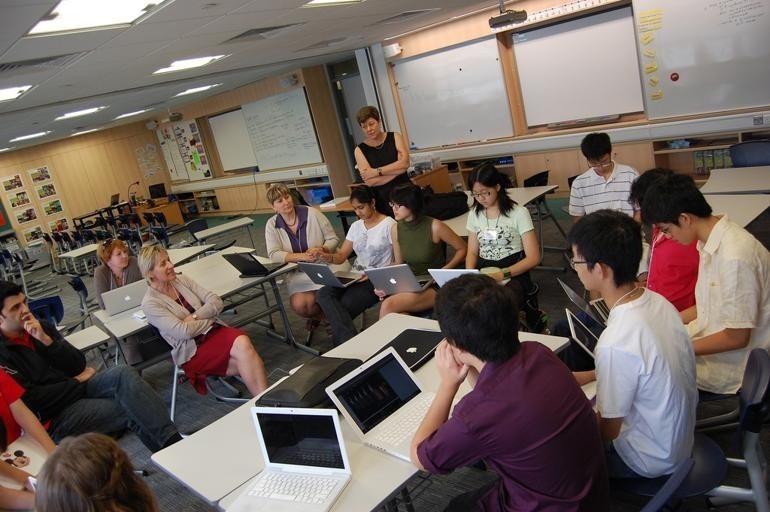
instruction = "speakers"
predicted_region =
[279,74,298,88]
[145,120,158,130]
[383,43,402,59]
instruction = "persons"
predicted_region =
[316,185,397,346]
[374,186,468,322]
[1,281,183,454]
[553,133,770,512]
[94,237,143,310]
[465,159,542,332]
[410,273,610,512]
[264,185,352,331]
[137,245,270,397]
[1,370,159,510]
[353,105,412,187]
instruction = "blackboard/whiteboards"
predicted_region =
[240,87,323,172]
[390,33,515,154]
[631,0,770,120]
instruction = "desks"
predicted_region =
[699,164,770,229]
[347,163,452,194]
[148,311,574,511]
[312,195,356,258]
[125,200,185,240]
[441,183,571,271]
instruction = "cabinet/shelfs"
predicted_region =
[458,153,521,190]
[577,142,656,176]
[653,128,770,182]
[512,150,582,192]
[171,165,353,217]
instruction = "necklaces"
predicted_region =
[113,270,123,281]
[365,134,387,149]
[364,217,386,230]
[483,205,503,229]
[285,213,297,226]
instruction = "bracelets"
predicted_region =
[501,267,512,280]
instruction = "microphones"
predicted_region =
[128,182,139,207]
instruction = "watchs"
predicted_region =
[377,166,383,177]
[191,312,199,321]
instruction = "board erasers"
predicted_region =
[409,147,417,150]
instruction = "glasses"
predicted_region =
[470,191,491,198]
[389,201,405,209]
[586,158,611,169]
[662,223,674,235]
[569,257,597,269]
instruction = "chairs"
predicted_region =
[40,211,236,278]
[640,456,696,512]
[634,347,769,512]
[523,170,568,264]
[730,140,770,167]
[0,241,119,371]
[145,323,245,422]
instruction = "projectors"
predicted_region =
[489,11,527,27]
[160,112,183,124]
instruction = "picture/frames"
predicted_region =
[1,165,69,245]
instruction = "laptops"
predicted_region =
[325,346,460,464]
[364,263,435,293]
[553,277,610,327]
[226,406,351,512]
[149,183,168,198]
[111,193,119,206]
[427,267,511,289]
[100,279,147,317]
[363,328,447,372]
[298,262,364,289]
[565,308,599,359]
[221,251,288,278]
